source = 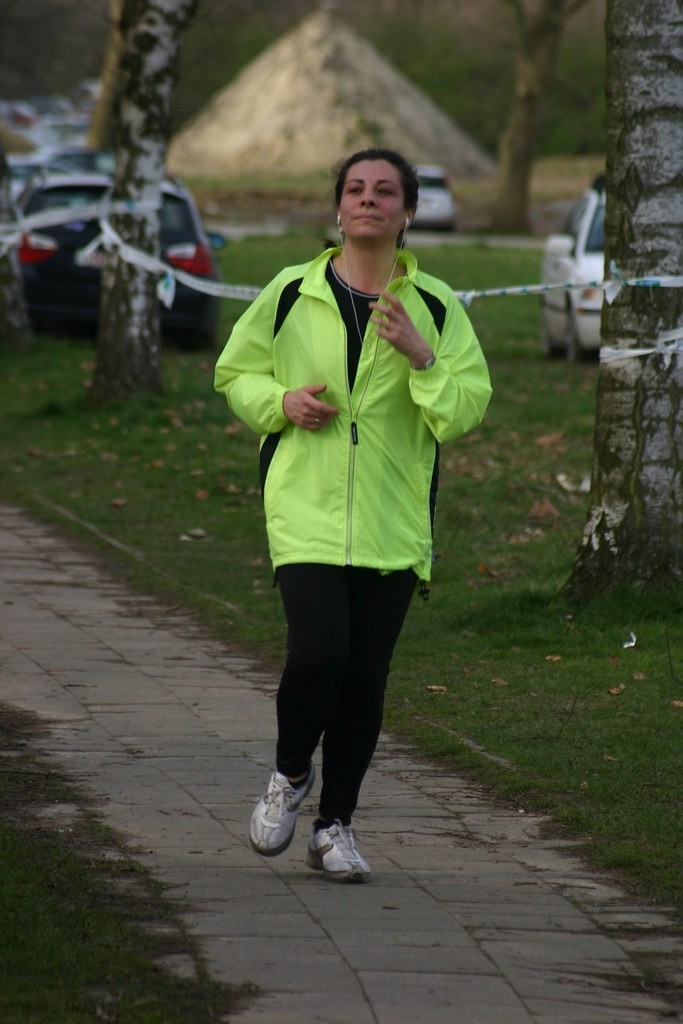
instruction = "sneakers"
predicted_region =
[304,817,371,883]
[249,757,316,857]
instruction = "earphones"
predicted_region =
[405,217,410,229]
[337,213,342,225]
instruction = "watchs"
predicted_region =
[410,351,436,371]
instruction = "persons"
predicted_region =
[212,150,492,883]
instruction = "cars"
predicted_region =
[4,166,228,347]
[408,164,459,232]
[536,174,611,366]
[0,78,121,182]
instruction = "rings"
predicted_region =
[313,417,319,425]
